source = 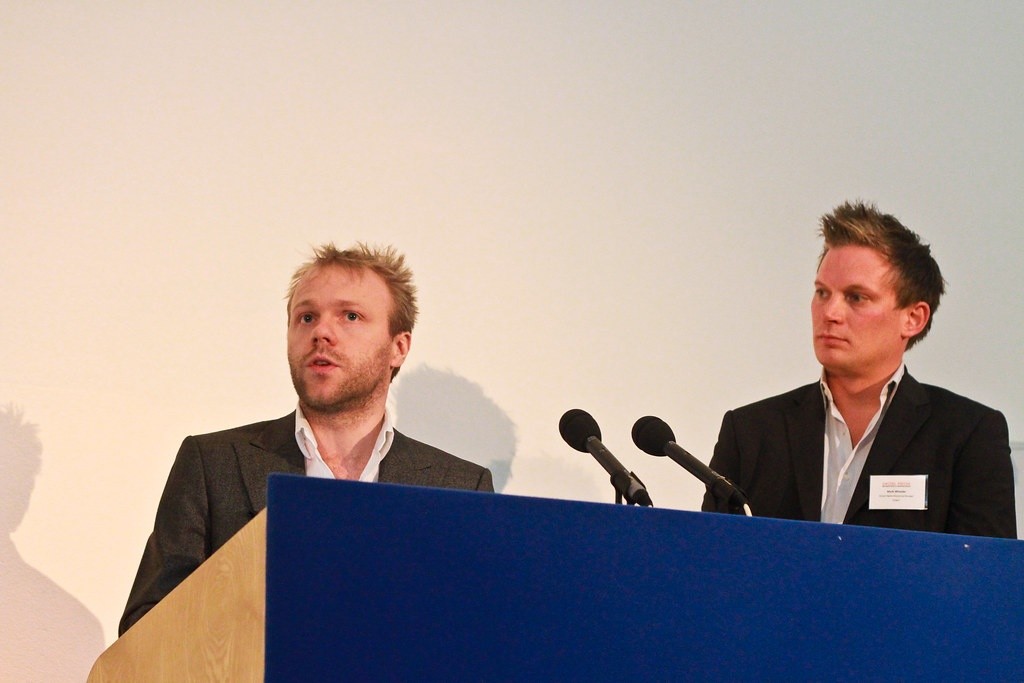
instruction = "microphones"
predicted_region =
[559,408,653,508]
[631,416,748,509]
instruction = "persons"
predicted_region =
[118,244,493,637]
[701,203,1018,538]
